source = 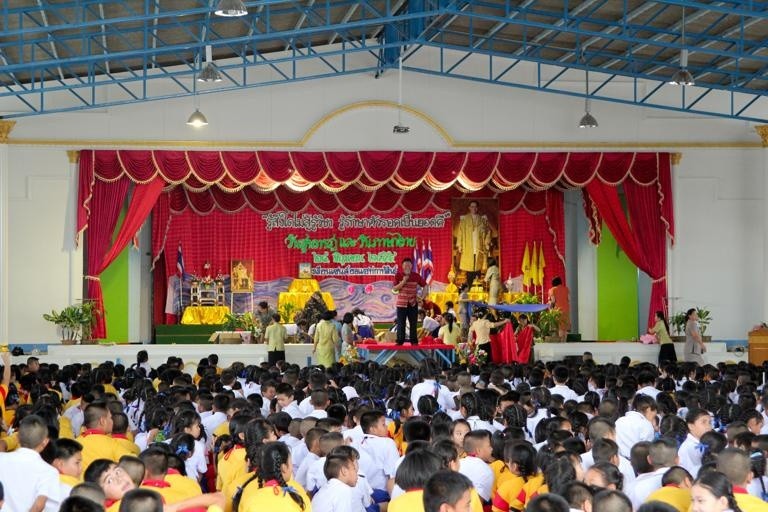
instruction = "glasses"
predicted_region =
[471,206,477,208]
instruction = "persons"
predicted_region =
[251,283,542,368]
[684,308,707,366]
[1,347,767,512]
[479,258,501,322]
[392,256,428,345]
[648,311,677,363]
[456,200,498,292]
[548,276,570,342]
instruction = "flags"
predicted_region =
[522,241,531,288]
[427,241,435,280]
[176,240,185,281]
[539,240,546,286]
[421,243,433,286]
[413,239,422,276]
[531,241,539,284]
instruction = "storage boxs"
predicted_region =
[233,330,253,344]
[208,332,243,344]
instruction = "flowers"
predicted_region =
[190,271,198,281]
[215,266,225,281]
[457,330,488,370]
[202,276,213,285]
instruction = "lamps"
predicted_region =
[668,6,697,88]
[213,0,249,18]
[195,61,224,82]
[186,45,210,131]
[579,50,599,131]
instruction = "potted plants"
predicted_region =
[538,306,569,343]
[667,311,687,342]
[234,316,244,332]
[82,300,104,345]
[224,319,234,331]
[696,306,713,341]
[275,302,300,335]
[42,307,88,345]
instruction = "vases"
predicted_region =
[204,283,209,290]
[191,282,197,287]
[216,282,222,286]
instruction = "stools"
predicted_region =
[230,291,255,317]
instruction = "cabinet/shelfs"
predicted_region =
[190,285,226,307]
[747,326,768,366]
